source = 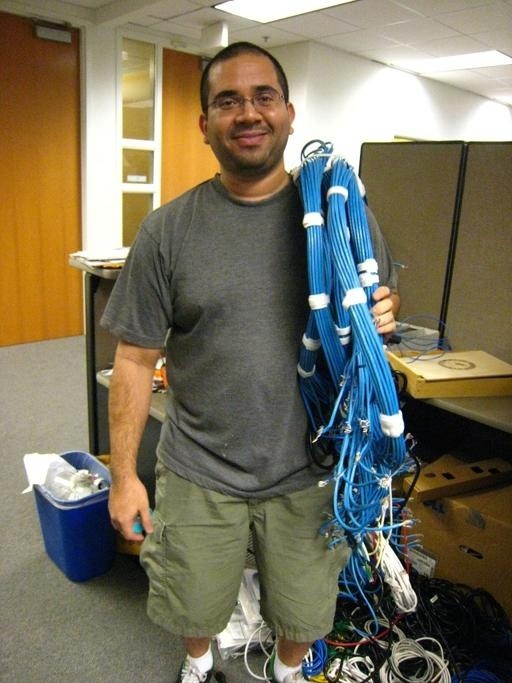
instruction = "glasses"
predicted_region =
[208,91,284,114]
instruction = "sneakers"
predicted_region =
[176,650,215,683]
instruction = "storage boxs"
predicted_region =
[404,451,512,617]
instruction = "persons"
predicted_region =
[100,41,402,682]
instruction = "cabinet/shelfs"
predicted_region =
[66,248,512,683]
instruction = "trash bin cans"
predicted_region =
[33,451,114,582]
